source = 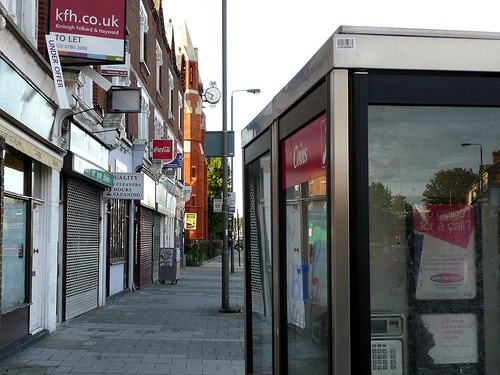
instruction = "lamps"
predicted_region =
[91,128,123,140]
[67,105,107,126]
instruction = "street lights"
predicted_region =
[461,143,484,202]
[230,89,261,273]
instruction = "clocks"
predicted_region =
[204,86,222,104]
